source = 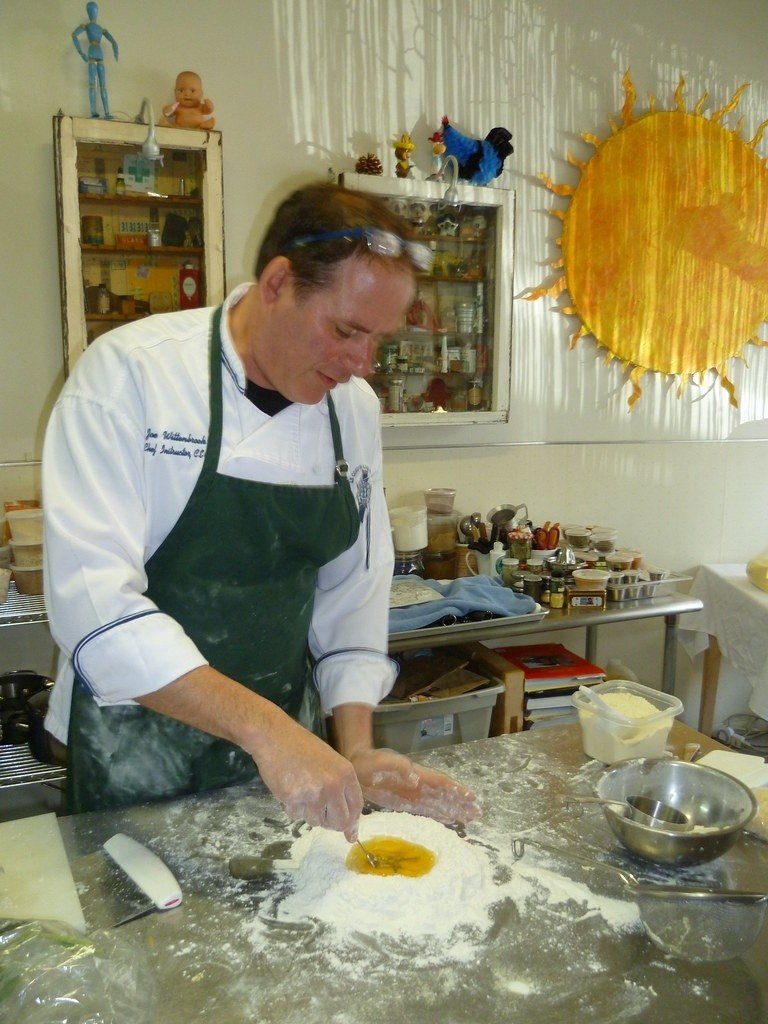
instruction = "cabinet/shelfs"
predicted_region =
[56,116,224,383]
[343,173,511,431]
[1,578,68,790]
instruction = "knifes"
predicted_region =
[99,831,184,929]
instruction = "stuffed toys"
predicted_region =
[428,133,446,174]
[393,135,415,177]
[438,115,514,186]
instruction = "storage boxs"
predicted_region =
[572,680,685,768]
[373,679,508,753]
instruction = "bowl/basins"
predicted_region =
[532,524,641,589]
[0,509,45,603]
[593,757,759,867]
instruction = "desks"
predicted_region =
[679,565,768,739]
[386,588,709,733]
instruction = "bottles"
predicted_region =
[179,178,186,196]
[394,550,477,580]
[387,380,404,413]
[83,215,103,244]
[148,208,161,246]
[116,168,125,195]
[468,379,483,410]
[448,343,487,377]
[383,344,412,373]
[455,301,475,333]
[490,537,567,607]
[97,284,111,315]
[180,263,200,311]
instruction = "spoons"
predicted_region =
[356,838,380,869]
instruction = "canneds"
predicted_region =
[82,215,104,245]
[383,344,484,414]
[501,559,575,608]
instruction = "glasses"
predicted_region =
[296,225,437,274]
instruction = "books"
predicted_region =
[494,643,607,731]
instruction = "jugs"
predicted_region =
[488,503,528,550]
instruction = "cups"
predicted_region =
[389,487,460,551]
[465,549,509,580]
[606,572,665,600]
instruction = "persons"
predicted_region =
[72,2,118,119]
[45,181,479,843]
[160,71,216,129]
